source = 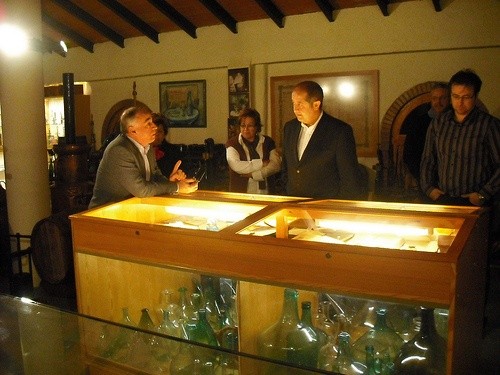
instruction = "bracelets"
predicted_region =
[479,194,485,202]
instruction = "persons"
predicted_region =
[88,106,222,208]
[226,109,281,194]
[419,69,500,206]
[402,83,449,180]
[282,81,361,200]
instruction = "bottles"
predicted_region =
[256,288,448,375]
[99,276,238,375]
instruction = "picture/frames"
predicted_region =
[158,80,207,129]
[270,69,380,158]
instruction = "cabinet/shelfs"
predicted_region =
[68,188,485,375]
